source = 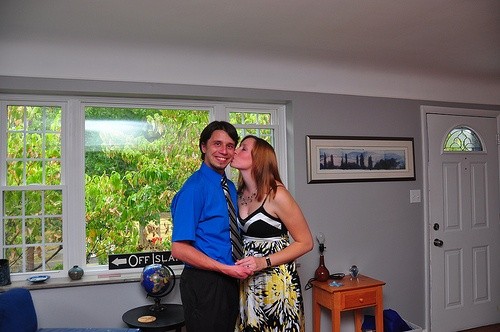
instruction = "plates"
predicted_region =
[27,275,49,282]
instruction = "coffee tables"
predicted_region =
[122,302,186,332]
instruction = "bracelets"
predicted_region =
[264,255,271,268]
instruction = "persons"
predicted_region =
[230,135,314,332]
[170,120,254,332]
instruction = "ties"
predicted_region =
[221,175,242,262]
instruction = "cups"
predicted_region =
[349,267,359,278]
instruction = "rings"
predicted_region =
[246,265,249,268]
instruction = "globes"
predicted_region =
[140,262,176,313]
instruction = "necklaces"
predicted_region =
[240,191,257,205]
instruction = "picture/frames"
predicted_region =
[305,134,416,184]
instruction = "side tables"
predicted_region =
[311,273,386,332]
